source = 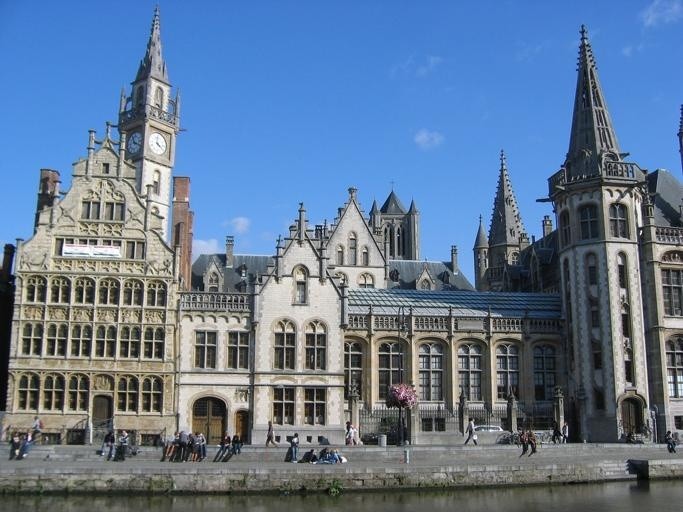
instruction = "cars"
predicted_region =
[361,422,406,446]
[472,425,504,432]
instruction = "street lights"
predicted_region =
[396,305,409,447]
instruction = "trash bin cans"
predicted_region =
[378,435,387,447]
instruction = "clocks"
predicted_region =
[126,130,143,154]
[148,131,167,156]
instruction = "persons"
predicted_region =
[7,415,43,459]
[265,421,278,448]
[100,431,116,457]
[290,433,299,463]
[520,420,677,454]
[120,430,130,461]
[220,431,231,457]
[345,421,358,446]
[307,447,341,464]
[232,432,242,455]
[159,430,206,462]
[464,417,478,445]
